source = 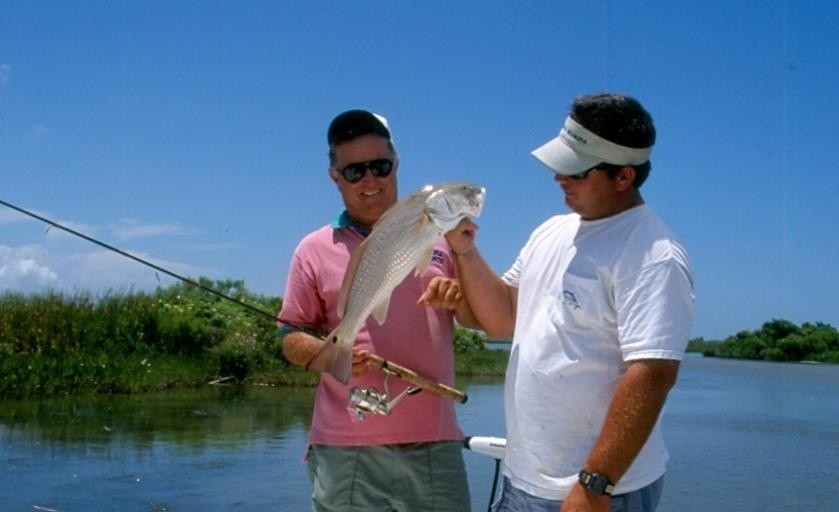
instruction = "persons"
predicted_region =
[278,110,488,512]
[443,93,699,512]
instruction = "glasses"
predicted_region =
[339,158,394,184]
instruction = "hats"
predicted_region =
[530,116,656,178]
[327,109,391,147]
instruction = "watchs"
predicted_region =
[579,467,616,497]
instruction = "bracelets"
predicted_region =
[454,249,474,259]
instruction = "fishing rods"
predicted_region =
[0,199,466,404]
